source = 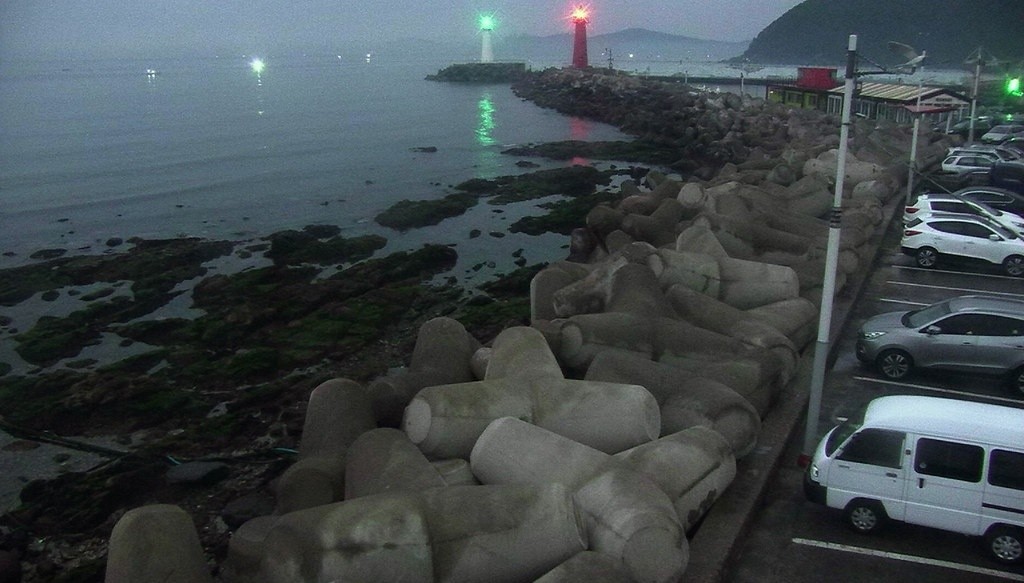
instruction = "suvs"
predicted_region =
[901,194,1023,228]
[898,213,1024,276]
[856,295,1024,396]
[981,125,1024,145]
[941,155,993,177]
[946,148,1017,163]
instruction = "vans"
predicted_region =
[805,392,1024,564]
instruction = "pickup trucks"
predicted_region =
[926,161,1024,197]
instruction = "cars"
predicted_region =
[951,187,1024,217]
